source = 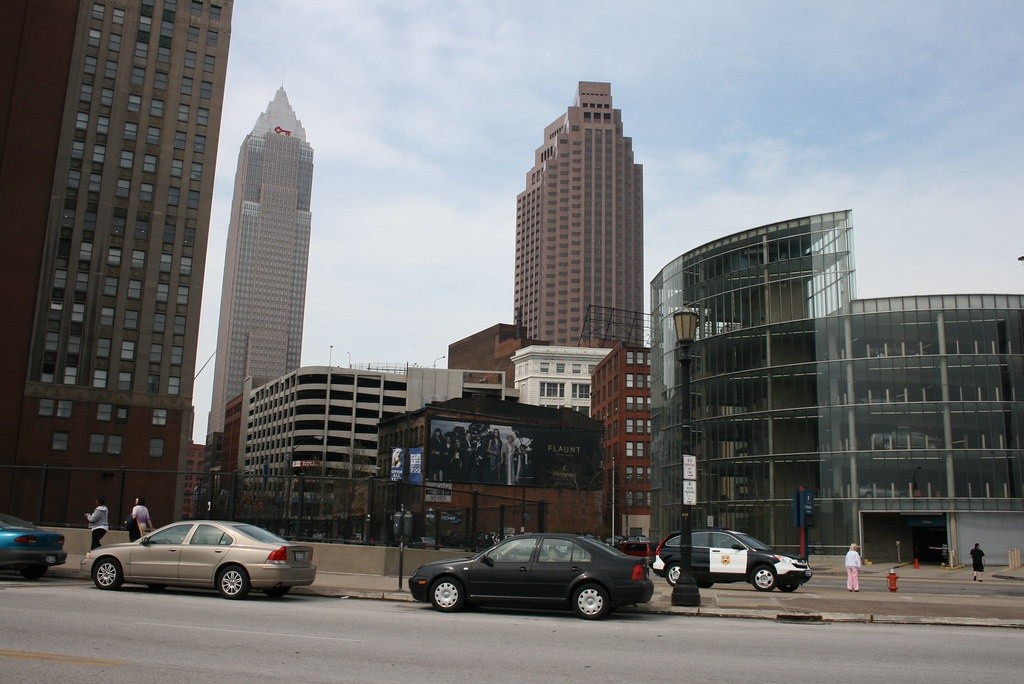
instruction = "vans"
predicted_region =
[613,542,658,567]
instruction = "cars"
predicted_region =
[407,536,443,549]
[0,513,68,581]
[80,520,317,600]
[407,534,655,621]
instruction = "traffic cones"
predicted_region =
[914,558,920,570]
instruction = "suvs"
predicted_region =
[652,528,814,593]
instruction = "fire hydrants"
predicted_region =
[886,567,900,593]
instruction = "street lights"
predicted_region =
[670,300,700,608]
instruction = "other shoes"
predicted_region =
[855,590,859,592]
[974,576,976,580]
[850,590,852,592]
[979,580,982,582]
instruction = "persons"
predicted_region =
[84,496,109,551]
[969,543,985,582]
[124,496,155,542]
[845,543,862,592]
